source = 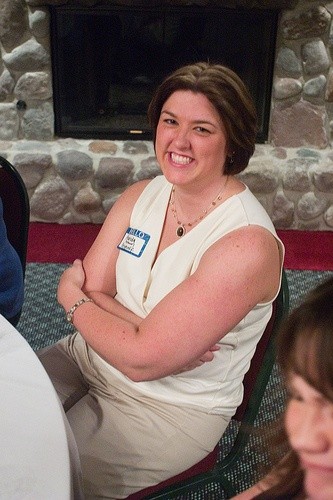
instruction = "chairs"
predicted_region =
[126,268,290,500]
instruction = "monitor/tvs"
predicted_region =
[49,4,280,143]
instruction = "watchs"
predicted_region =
[66,297,93,324]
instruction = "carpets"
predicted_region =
[13,263,333,500]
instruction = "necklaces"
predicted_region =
[169,175,229,238]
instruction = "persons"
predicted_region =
[246,274,333,500]
[35,62,285,500]
[0,198,24,327]
